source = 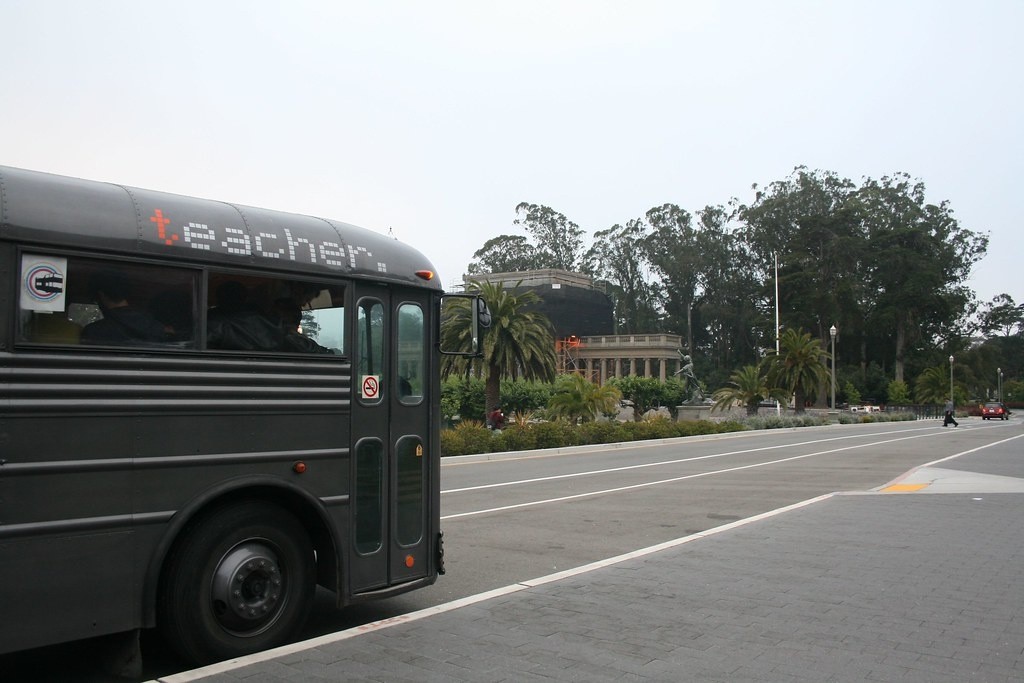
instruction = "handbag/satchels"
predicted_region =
[945,414,952,423]
[487,413,498,431]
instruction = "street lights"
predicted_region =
[997,368,1001,401]
[948,355,955,405]
[1000,372,1003,402]
[830,324,837,410]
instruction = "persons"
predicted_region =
[672,348,705,402]
[24,274,333,356]
[942,398,959,427]
[488,404,505,430]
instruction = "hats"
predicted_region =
[267,297,303,324]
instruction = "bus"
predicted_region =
[0,167,493,683]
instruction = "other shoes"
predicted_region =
[955,423,958,427]
[943,425,947,426]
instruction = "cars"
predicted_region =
[982,401,1009,420]
